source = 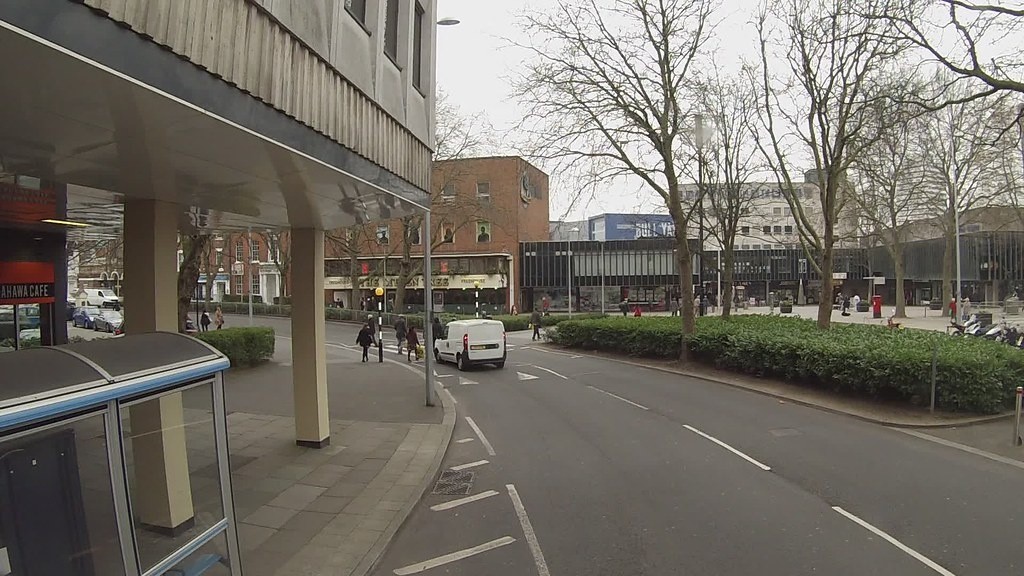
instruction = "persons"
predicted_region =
[541,298,549,316]
[530,308,542,341]
[619,299,631,315]
[671,298,682,317]
[962,298,970,321]
[386,298,394,312]
[510,305,517,316]
[336,298,341,308]
[633,304,642,317]
[200,309,210,332]
[359,296,383,312]
[393,314,409,356]
[703,295,709,316]
[406,326,420,362]
[356,322,373,363]
[215,306,225,330]
[837,293,850,314]
[113,302,120,311]
[854,294,860,313]
[948,298,957,320]
[367,315,379,347]
[694,295,701,318]
[433,317,443,348]
[733,294,740,312]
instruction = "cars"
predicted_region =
[72,306,99,329]
[92,310,124,333]
[18,306,40,329]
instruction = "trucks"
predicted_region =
[84,289,119,308]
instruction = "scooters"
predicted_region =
[950,314,1024,350]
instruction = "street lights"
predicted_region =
[597,239,607,314]
[375,287,384,363]
[473,281,480,318]
[566,227,579,320]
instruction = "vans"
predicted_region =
[433,319,507,372]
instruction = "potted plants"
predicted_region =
[857,301,870,312]
[929,301,943,310]
[780,301,792,313]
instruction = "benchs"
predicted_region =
[169,553,222,576]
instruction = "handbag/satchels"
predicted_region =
[948,309,953,316]
[962,315,968,320]
[417,345,425,358]
